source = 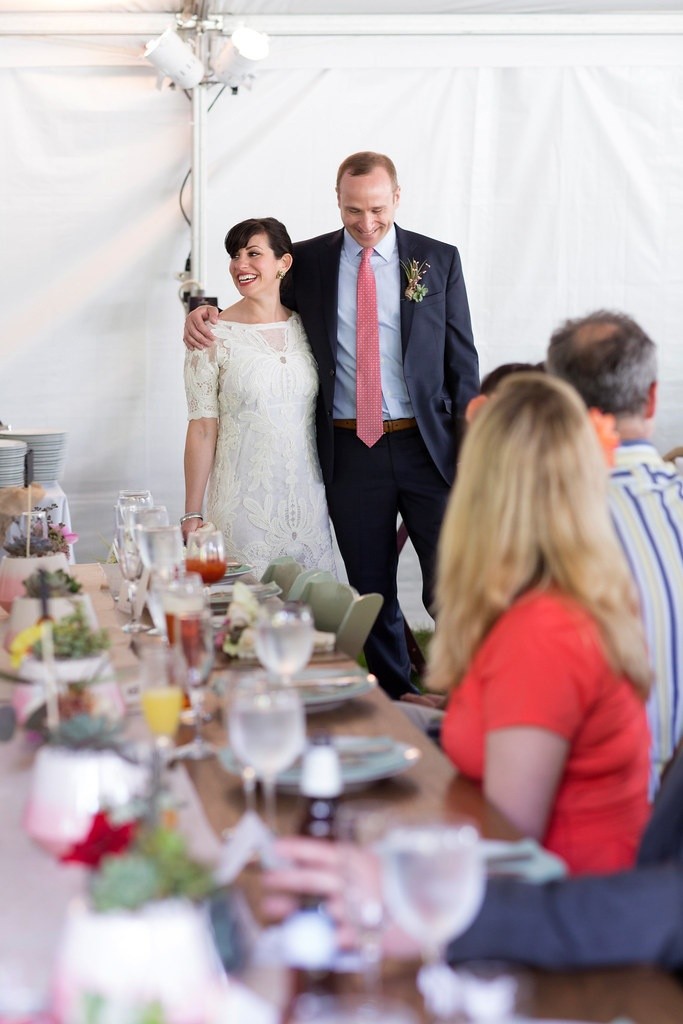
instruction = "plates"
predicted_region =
[0,429,67,488]
[220,562,257,584]
[222,736,422,790]
[205,585,282,611]
[214,668,377,713]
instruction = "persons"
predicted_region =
[547,313,683,805]
[182,217,340,621]
[469,363,546,428]
[184,152,480,701]
[259,741,683,994]
[440,373,650,875]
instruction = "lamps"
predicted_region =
[143,27,205,89]
[209,24,271,88]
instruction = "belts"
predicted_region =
[334,416,418,432]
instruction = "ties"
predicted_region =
[355,247,384,447]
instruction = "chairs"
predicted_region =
[294,571,383,659]
[260,555,317,601]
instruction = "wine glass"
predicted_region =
[109,488,487,1012]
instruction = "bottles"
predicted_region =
[299,734,342,840]
[280,894,338,1023]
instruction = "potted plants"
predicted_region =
[6,569,230,1024]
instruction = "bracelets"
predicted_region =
[180,512,202,523]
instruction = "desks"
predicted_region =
[0,557,683,1024]
